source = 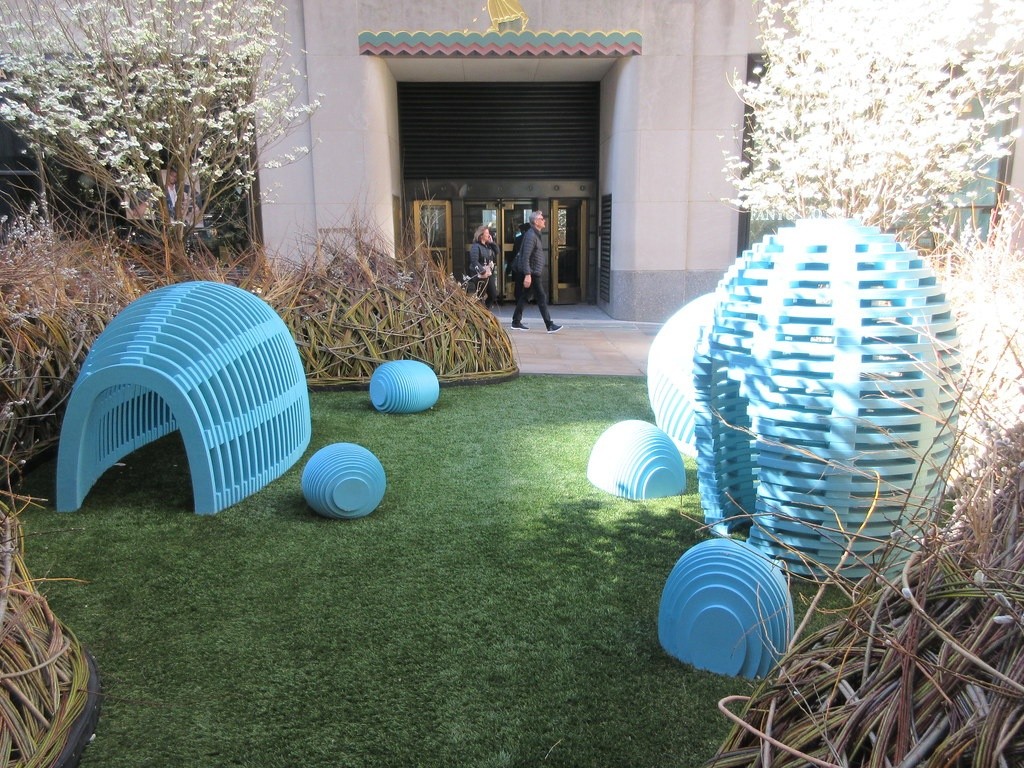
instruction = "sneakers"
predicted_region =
[511,321,530,331]
[547,324,563,333]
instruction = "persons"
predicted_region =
[158,167,201,224]
[469,225,500,310]
[507,210,563,333]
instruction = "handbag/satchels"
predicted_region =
[478,266,492,278]
